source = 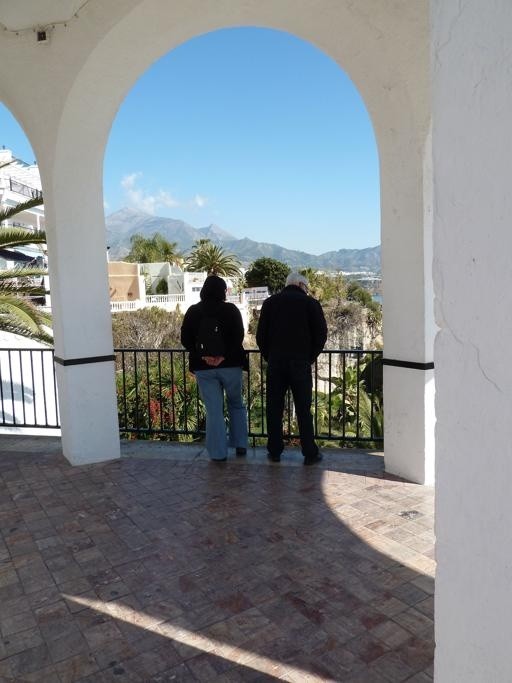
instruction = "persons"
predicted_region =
[181,276,250,462]
[256,273,328,465]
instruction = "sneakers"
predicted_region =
[304,454,323,464]
[212,457,228,461]
[268,452,281,462]
[235,447,247,458]
